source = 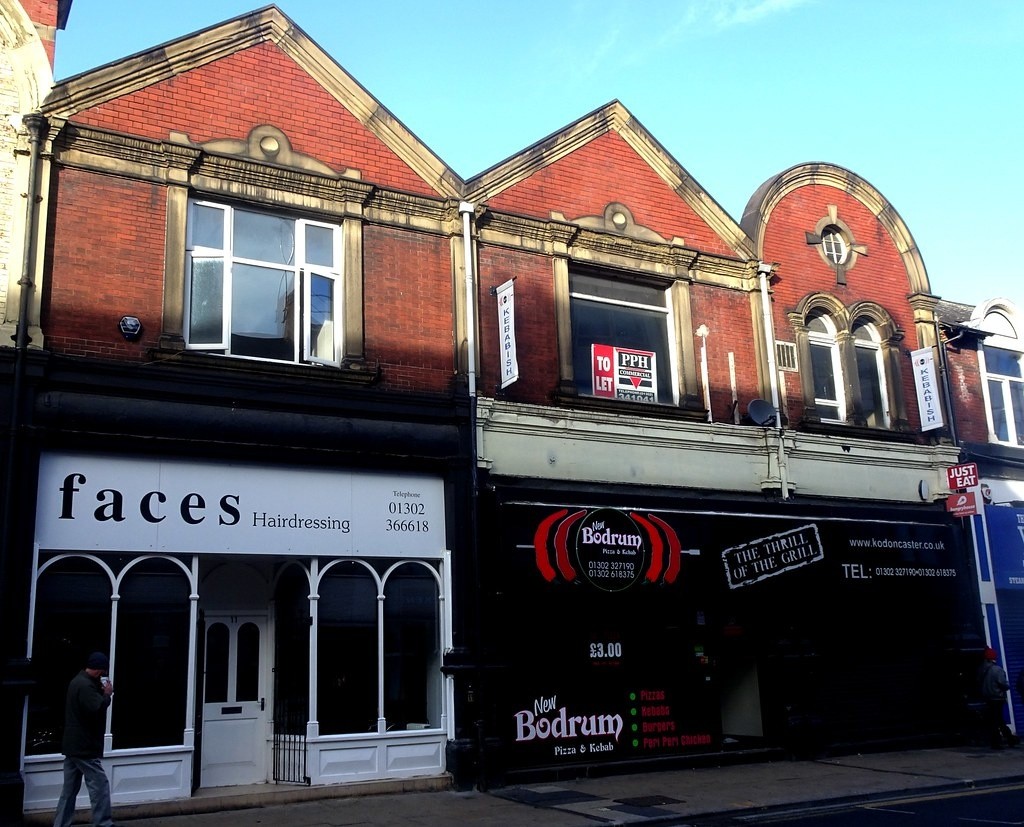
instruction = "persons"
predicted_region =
[977,650,1020,748]
[1015,668,1024,705]
[54,653,124,827]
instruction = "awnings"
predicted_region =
[482,476,979,603]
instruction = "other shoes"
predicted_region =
[992,742,1005,750]
[1008,735,1021,747]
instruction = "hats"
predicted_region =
[87,652,109,670]
[984,648,997,659]
